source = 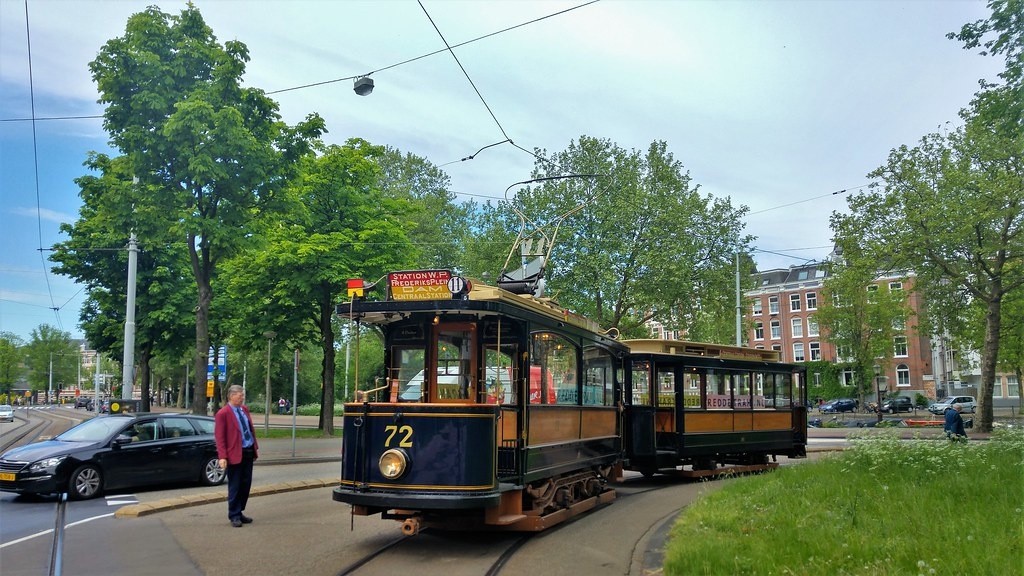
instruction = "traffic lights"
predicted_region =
[58,382,63,390]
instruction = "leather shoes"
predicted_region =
[231,515,253,527]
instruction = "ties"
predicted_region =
[237,408,250,441]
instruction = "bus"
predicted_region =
[330,266,809,536]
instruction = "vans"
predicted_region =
[397,362,557,405]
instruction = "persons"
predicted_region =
[131,421,151,442]
[943,402,969,445]
[5,395,8,405]
[818,400,823,413]
[822,399,826,405]
[17,396,23,406]
[278,397,285,415]
[149,394,154,406]
[170,428,181,438]
[285,399,291,414]
[15,395,19,406]
[214,385,259,528]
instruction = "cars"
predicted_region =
[74,398,91,409]
[0,412,228,501]
[86,399,103,411]
[0,404,15,422]
[929,396,977,416]
[99,402,109,414]
[877,396,913,414]
[792,397,813,412]
[819,398,858,415]
